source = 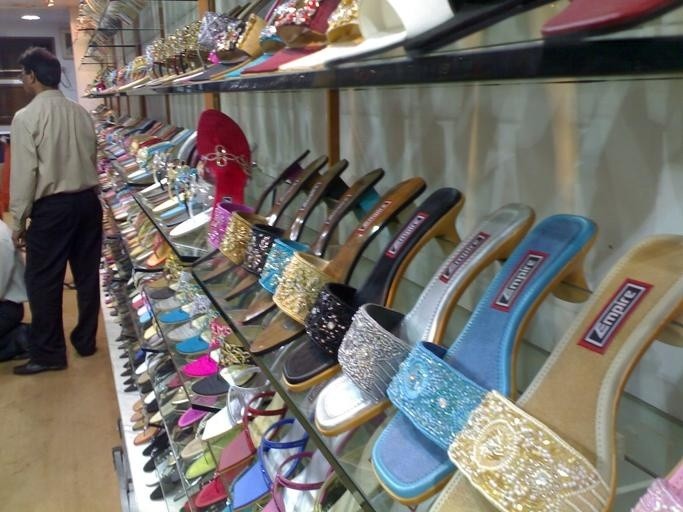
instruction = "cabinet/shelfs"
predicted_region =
[76,0,683,511]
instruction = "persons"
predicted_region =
[6,46,103,374]
[0,219,32,361]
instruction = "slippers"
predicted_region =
[74,0,683,512]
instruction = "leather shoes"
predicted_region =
[13,359,68,375]
